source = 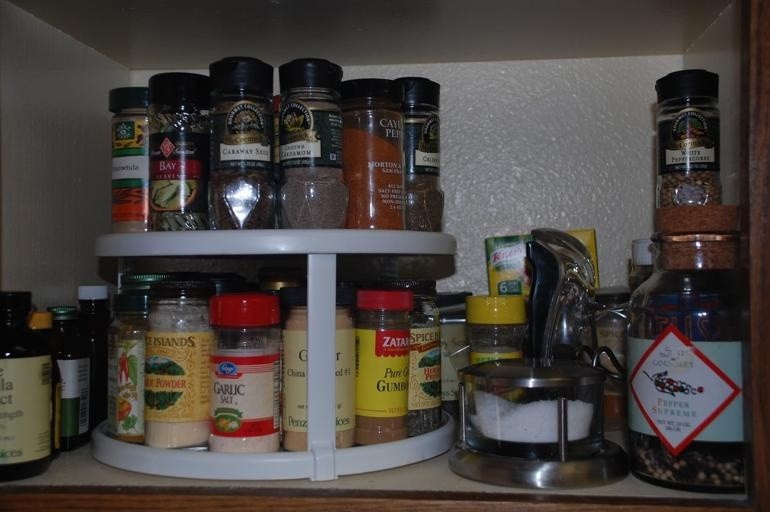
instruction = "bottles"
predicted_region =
[595,286,630,431]
[208,57,274,229]
[282,287,355,451]
[26,312,54,342]
[278,58,347,228]
[207,294,282,452]
[147,72,208,231]
[122,274,172,292]
[274,96,279,227]
[386,280,441,437]
[146,277,214,448]
[109,87,149,232]
[107,292,146,444]
[355,290,412,445]
[398,77,444,232]
[262,274,308,294]
[79,286,109,427]
[438,291,473,419]
[0,292,53,479]
[211,273,246,294]
[340,79,404,229]
[628,206,751,491]
[655,68,720,205]
[48,306,95,449]
[466,295,527,367]
[627,240,654,294]
